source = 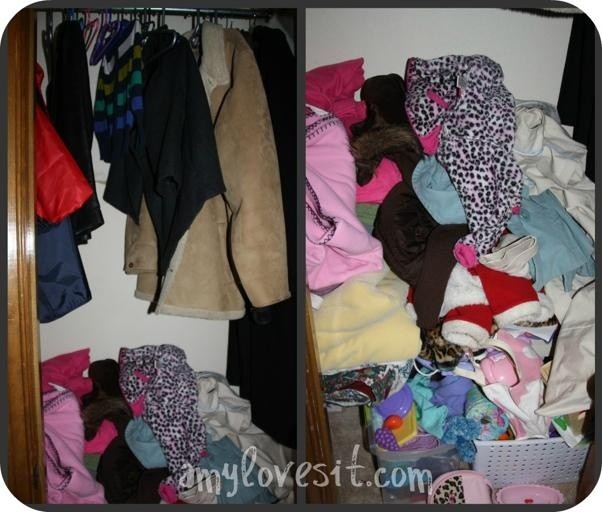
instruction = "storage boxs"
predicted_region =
[363,404,471,504]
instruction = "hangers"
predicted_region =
[42,7,256,66]
